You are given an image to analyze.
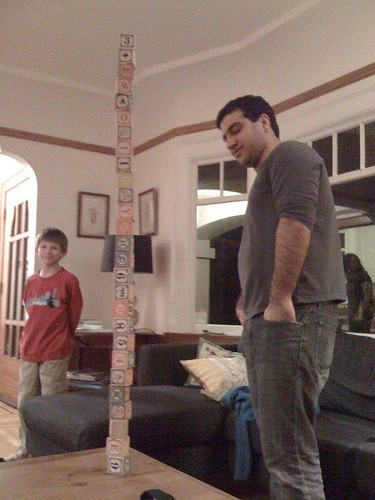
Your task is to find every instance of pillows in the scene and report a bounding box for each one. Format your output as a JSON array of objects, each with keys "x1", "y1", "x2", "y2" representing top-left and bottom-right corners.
[
  {"x1": 184, "y1": 336, "x2": 244, "y2": 387},
  {"x1": 179, "y1": 357, "x2": 249, "y2": 401}
]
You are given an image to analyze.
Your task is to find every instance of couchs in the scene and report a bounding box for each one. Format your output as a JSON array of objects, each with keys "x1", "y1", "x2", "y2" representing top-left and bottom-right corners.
[{"x1": 20, "y1": 330, "x2": 375, "y2": 500}]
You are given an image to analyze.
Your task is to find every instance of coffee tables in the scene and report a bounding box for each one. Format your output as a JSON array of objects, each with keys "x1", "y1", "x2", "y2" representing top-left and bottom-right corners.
[{"x1": 0, "y1": 446, "x2": 243, "y2": 500}]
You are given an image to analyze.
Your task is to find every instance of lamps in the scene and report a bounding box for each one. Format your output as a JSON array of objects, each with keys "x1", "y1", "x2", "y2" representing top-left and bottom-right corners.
[{"x1": 99, "y1": 236, "x2": 153, "y2": 325}]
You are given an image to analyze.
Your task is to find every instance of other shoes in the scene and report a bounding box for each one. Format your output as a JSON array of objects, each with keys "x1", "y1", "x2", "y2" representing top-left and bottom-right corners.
[{"x1": 0, "y1": 451, "x2": 28, "y2": 462}]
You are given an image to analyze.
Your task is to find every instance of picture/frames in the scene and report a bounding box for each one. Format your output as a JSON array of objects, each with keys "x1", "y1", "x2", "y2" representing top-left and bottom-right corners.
[
  {"x1": 138, "y1": 187, "x2": 159, "y2": 237},
  {"x1": 77, "y1": 191, "x2": 111, "y2": 239}
]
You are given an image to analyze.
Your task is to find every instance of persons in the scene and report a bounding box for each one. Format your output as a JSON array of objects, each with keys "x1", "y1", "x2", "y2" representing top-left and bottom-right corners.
[
  {"x1": 0, "y1": 227, "x2": 84, "y2": 462},
  {"x1": 216, "y1": 95, "x2": 375, "y2": 500}
]
